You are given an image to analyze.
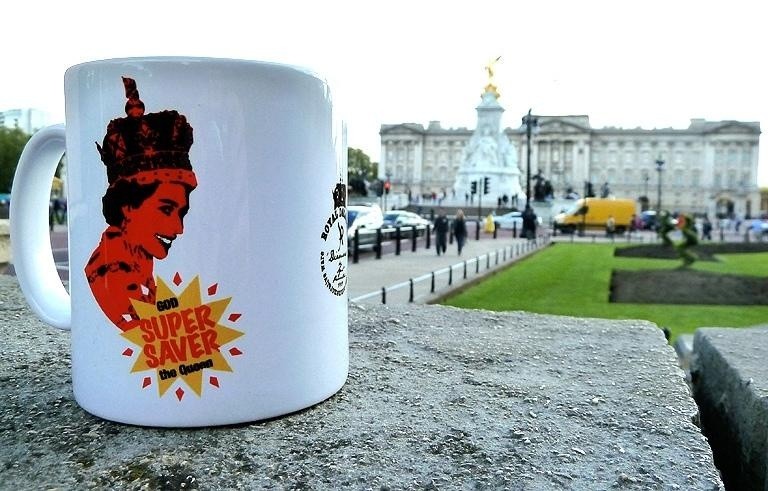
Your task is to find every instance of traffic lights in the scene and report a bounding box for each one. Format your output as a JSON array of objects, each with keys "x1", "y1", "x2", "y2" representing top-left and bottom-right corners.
[
  {"x1": 384, "y1": 180, "x2": 391, "y2": 194},
  {"x1": 471, "y1": 180, "x2": 477, "y2": 193},
  {"x1": 484, "y1": 177, "x2": 491, "y2": 194}
]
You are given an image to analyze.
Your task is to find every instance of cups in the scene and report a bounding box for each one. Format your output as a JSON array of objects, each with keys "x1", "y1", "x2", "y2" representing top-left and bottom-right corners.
[{"x1": 1, "y1": 50, "x2": 353, "y2": 432}]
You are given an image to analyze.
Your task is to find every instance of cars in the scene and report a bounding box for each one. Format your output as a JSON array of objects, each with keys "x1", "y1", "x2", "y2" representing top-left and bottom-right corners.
[
  {"x1": 640, "y1": 210, "x2": 674, "y2": 231},
  {"x1": 484, "y1": 210, "x2": 542, "y2": 230},
  {"x1": 384, "y1": 211, "x2": 431, "y2": 240}
]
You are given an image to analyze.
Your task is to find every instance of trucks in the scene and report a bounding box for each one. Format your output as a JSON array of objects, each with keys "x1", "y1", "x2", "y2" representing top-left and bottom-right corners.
[{"x1": 552, "y1": 197, "x2": 636, "y2": 234}]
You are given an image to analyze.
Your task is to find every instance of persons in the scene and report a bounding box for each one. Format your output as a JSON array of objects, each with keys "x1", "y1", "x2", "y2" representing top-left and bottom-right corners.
[
  {"x1": 452, "y1": 207, "x2": 468, "y2": 257},
  {"x1": 83, "y1": 77, "x2": 200, "y2": 335},
  {"x1": 430, "y1": 206, "x2": 449, "y2": 256},
  {"x1": 552, "y1": 208, "x2": 767, "y2": 244},
  {"x1": 518, "y1": 202, "x2": 536, "y2": 243},
  {"x1": 483, "y1": 208, "x2": 496, "y2": 237}
]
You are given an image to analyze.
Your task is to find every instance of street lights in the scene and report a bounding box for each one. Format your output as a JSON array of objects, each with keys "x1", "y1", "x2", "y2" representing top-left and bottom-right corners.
[
  {"x1": 519, "y1": 110, "x2": 541, "y2": 237},
  {"x1": 654, "y1": 154, "x2": 663, "y2": 210}
]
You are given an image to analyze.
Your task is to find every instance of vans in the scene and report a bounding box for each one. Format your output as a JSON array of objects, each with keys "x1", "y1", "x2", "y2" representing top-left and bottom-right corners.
[{"x1": 347, "y1": 203, "x2": 384, "y2": 255}]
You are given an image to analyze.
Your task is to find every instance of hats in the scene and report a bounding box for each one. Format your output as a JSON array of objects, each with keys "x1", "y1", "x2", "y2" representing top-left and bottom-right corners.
[{"x1": 103, "y1": 109, "x2": 199, "y2": 190}]
